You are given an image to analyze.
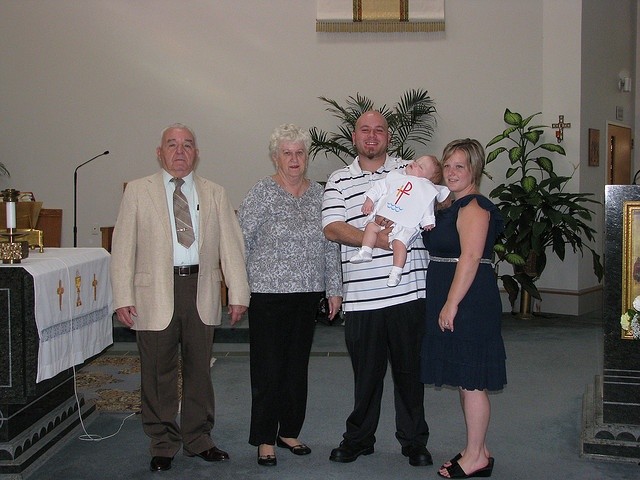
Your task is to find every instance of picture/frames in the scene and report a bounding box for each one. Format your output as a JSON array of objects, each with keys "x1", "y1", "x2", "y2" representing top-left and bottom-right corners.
[{"x1": 588, "y1": 127, "x2": 600, "y2": 167}]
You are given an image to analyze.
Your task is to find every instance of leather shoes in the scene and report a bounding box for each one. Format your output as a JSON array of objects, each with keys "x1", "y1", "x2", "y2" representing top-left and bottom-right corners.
[
  {"x1": 400, "y1": 443, "x2": 434, "y2": 468},
  {"x1": 329, "y1": 436, "x2": 377, "y2": 464},
  {"x1": 276, "y1": 434, "x2": 312, "y2": 456},
  {"x1": 149, "y1": 455, "x2": 174, "y2": 471},
  {"x1": 256, "y1": 443, "x2": 277, "y2": 467},
  {"x1": 181, "y1": 438, "x2": 229, "y2": 462}
]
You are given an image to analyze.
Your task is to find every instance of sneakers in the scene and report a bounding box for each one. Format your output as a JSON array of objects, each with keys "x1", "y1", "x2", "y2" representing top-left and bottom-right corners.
[
  {"x1": 350, "y1": 246, "x2": 374, "y2": 264},
  {"x1": 386, "y1": 266, "x2": 403, "y2": 287}
]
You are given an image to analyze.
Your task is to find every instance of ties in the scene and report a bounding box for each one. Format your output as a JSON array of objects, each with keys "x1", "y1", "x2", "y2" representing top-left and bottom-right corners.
[{"x1": 167, "y1": 177, "x2": 195, "y2": 248}]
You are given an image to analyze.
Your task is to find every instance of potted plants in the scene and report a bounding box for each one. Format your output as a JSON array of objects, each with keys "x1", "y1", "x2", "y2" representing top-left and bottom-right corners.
[{"x1": 486, "y1": 108, "x2": 605, "y2": 310}]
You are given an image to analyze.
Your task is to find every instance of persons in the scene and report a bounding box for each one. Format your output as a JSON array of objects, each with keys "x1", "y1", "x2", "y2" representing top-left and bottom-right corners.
[
  {"x1": 418, "y1": 136, "x2": 509, "y2": 480},
  {"x1": 108, "y1": 124, "x2": 252, "y2": 473},
  {"x1": 321, "y1": 109, "x2": 440, "y2": 467},
  {"x1": 348, "y1": 154, "x2": 442, "y2": 288},
  {"x1": 235, "y1": 121, "x2": 345, "y2": 467}
]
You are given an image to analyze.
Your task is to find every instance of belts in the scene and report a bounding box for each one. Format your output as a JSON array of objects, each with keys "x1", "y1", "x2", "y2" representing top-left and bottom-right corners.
[{"x1": 173, "y1": 263, "x2": 200, "y2": 278}]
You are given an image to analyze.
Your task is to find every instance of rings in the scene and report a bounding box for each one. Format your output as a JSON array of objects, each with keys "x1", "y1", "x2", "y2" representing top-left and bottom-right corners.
[{"x1": 337, "y1": 307, "x2": 341, "y2": 312}]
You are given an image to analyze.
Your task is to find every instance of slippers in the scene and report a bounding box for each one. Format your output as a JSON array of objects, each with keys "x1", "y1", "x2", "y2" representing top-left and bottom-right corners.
[
  {"x1": 441, "y1": 450, "x2": 494, "y2": 469},
  {"x1": 436, "y1": 459, "x2": 493, "y2": 477}
]
